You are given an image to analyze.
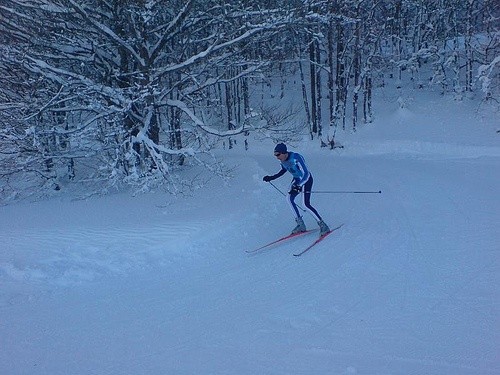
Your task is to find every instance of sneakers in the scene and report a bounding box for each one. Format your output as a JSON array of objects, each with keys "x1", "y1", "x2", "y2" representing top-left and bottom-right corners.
[
  {"x1": 318, "y1": 219, "x2": 331, "y2": 236},
  {"x1": 291, "y1": 217, "x2": 306, "y2": 234}
]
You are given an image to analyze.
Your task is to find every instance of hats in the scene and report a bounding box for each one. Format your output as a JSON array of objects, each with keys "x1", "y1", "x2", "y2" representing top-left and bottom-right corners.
[{"x1": 274, "y1": 142, "x2": 287, "y2": 153}]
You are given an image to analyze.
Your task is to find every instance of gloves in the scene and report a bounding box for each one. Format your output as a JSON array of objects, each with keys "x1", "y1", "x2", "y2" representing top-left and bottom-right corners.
[
  {"x1": 289, "y1": 187, "x2": 300, "y2": 196},
  {"x1": 263, "y1": 175, "x2": 271, "y2": 182}
]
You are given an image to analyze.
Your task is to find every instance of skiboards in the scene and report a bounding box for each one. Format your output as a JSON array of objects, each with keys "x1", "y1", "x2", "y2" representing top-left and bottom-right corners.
[{"x1": 244, "y1": 223, "x2": 345, "y2": 257}]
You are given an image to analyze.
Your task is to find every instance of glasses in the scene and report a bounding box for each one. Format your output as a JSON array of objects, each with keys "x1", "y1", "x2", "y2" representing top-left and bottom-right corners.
[{"x1": 275, "y1": 153, "x2": 282, "y2": 157}]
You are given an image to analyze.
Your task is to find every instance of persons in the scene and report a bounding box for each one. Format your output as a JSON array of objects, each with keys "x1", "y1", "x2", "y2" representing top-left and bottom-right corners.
[{"x1": 262, "y1": 142, "x2": 331, "y2": 239}]
